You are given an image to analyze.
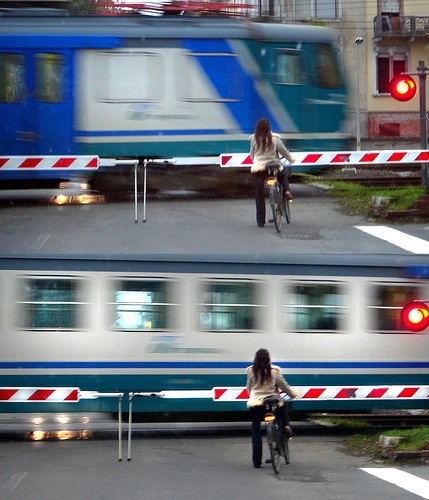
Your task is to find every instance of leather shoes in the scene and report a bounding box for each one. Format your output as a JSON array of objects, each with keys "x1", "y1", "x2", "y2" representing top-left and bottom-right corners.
[
  {"x1": 286, "y1": 194, "x2": 293, "y2": 199},
  {"x1": 285, "y1": 426, "x2": 293, "y2": 437},
  {"x1": 258, "y1": 222, "x2": 263, "y2": 227},
  {"x1": 254, "y1": 462, "x2": 261, "y2": 468}
]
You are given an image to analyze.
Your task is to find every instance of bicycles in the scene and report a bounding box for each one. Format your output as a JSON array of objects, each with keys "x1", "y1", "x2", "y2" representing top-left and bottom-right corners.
[
  {"x1": 264, "y1": 156, "x2": 291, "y2": 232},
  {"x1": 264, "y1": 388, "x2": 291, "y2": 474}
]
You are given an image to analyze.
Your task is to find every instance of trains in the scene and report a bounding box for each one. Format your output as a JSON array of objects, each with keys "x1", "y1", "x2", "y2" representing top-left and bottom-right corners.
[
  {"x1": 1, "y1": 1, "x2": 352, "y2": 197},
  {"x1": 0, "y1": 247, "x2": 429, "y2": 431}
]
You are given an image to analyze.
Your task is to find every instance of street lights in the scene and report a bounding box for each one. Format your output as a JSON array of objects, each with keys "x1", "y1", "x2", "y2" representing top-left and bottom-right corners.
[{"x1": 354, "y1": 37, "x2": 364, "y2": 152}]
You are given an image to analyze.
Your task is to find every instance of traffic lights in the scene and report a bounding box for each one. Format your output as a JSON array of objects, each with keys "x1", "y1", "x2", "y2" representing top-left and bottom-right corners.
[
  {"x1": 390, "y1": 73, "x2": 416, "y2": 102},
  {"x1": 402, "y1": 302, "x2": 428, "y2": 331}
]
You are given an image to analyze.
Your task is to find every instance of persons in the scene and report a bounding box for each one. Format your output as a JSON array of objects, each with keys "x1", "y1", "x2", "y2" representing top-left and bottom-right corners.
[
  {"x1": 246, "y1": 349, "x2": 297, "y2": 468},
  {"x1": 249, "y1": 118, "x2": 296, "y2": 227}
]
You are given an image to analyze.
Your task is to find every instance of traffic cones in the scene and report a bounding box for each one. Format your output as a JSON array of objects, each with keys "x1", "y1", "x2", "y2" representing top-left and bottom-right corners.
[
  {"x1": 0, "y1": 384, "x2": 429, "y2": 463},
  {"x1": 0, "y1": 150, "x2": 429, "y2": 222}
]
